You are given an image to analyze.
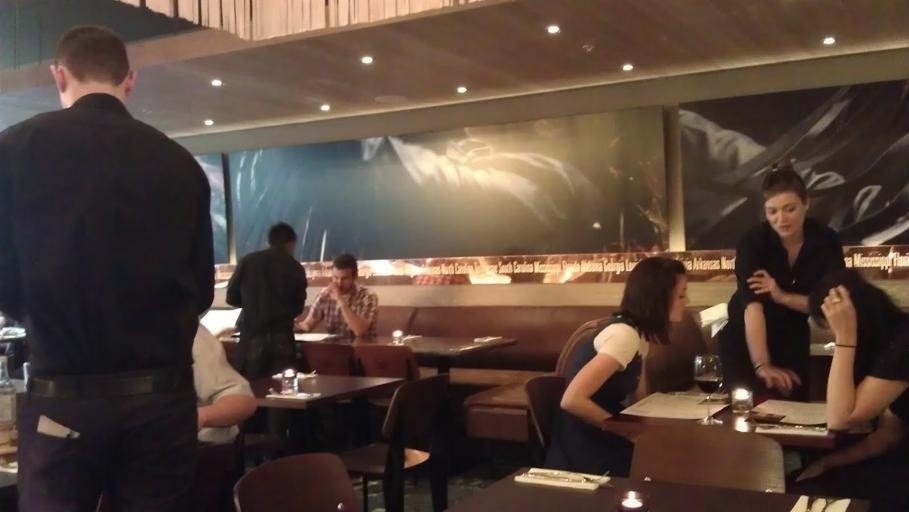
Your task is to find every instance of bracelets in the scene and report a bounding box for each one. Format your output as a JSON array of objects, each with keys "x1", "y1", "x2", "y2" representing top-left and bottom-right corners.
[
  {"x1": 752, "y1": 360, "x2": 770, "y2": 372},
  {"x1": 835, "y1": 342, "x2": 857, "y2": 349}
]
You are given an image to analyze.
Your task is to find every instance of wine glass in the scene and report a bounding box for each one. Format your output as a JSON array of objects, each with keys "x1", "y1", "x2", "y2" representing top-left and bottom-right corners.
[{"x1": 693, "y1": 353, "x2": 728, "y2": 429}]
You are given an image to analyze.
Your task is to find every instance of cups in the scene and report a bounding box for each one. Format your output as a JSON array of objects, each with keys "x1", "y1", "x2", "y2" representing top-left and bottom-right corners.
[
  {"x1": 613, "y1": 491, "x2": 648, "y2": 511},
  {"x1": 731, "y1": 390, "x2": 755, "y2": 416},
  {"x1": 280, "y1": 372, "x2": 299, "y2": 396}
]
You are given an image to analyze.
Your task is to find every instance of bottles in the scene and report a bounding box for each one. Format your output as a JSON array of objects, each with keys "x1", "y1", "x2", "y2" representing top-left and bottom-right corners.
[{"x1": 0, "y1": 356, "x2": 20, "y2": 469}]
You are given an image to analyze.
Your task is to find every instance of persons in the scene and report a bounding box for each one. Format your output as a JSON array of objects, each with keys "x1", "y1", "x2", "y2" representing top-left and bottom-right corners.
[
  {"x1": 226, "y1": 221, "x2": 308, "y2": 454},
  {"x1": 0, "y1": 24, "x2": 215, "y2": 511},
  {"x1": 193, "y1": 316, "x2": 256, "y2": 447},
  {"x1": 717, "y1": 162, "x2": 844, "y2": 393},
  {"x1": 785, "y1": 270, "x2": 905, "y2": 498},
  {"x1": 292, "y1": 254, "x2": 378, "y2": 337},
  {"x1": 557, "y1": 252, "x2": 688, "y2": 472}
]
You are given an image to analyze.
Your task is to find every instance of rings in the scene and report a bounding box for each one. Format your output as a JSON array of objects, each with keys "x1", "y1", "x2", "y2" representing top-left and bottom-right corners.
[{"x1": 831, "y1": 298, "x2": 840, "y2": 303}]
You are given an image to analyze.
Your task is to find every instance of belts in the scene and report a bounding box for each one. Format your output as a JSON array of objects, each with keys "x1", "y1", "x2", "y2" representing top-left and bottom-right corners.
[{"x1": 21, "y1": 371, "x2": 197, "y2": 401}]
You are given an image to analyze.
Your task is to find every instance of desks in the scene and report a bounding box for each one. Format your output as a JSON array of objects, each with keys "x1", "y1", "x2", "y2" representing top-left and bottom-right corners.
[{"x1": 0, "y1": 301, "x2": 909, "y2": 512}]
